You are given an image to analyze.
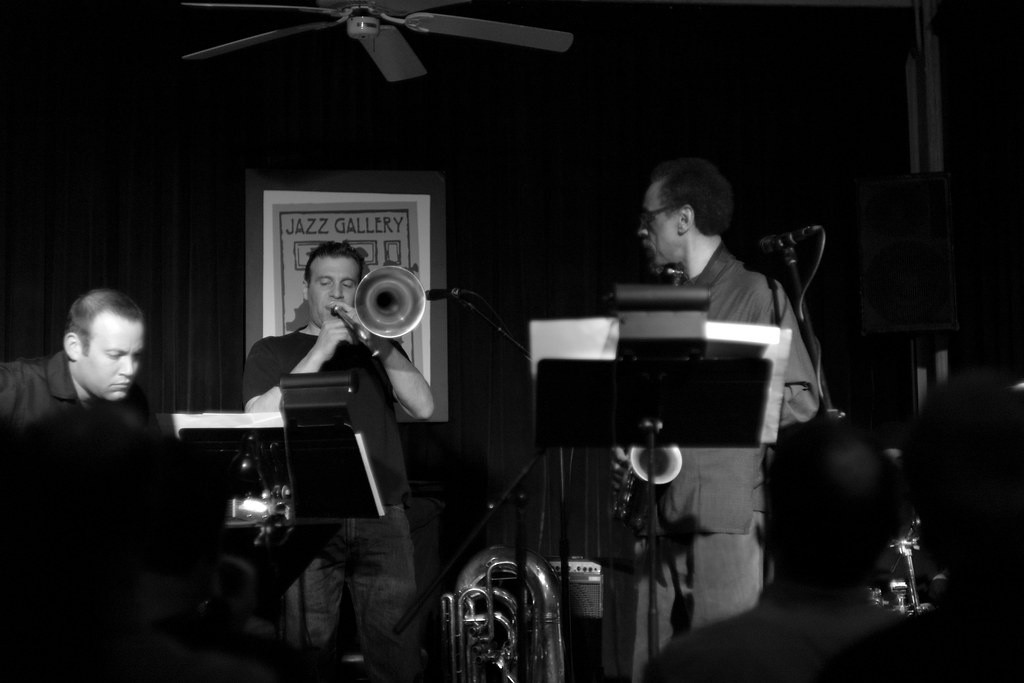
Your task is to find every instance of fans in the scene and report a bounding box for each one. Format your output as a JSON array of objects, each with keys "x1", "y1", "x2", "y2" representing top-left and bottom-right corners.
[{"x1": 178, "y1": 0, "x2": 575, "y2": 83}]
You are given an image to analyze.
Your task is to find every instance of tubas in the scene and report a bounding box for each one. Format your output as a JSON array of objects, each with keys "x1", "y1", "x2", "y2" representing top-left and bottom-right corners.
[{"x1": 439, "y1": 543, "x2": 568, "y2": 683}]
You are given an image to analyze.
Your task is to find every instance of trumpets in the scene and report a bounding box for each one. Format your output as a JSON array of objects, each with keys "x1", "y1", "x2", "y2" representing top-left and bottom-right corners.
[{"x1": 325, "y1": 263, "x2": 427, "y2": 358}]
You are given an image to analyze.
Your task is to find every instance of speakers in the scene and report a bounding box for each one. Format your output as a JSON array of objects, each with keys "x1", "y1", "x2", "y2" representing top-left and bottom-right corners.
[{"x1": 537, "y1": 556, "x2": 605, "y2": 683}]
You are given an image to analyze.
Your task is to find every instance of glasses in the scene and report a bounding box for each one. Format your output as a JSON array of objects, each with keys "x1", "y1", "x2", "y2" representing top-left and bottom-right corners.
[{"x1": 635, "y1": 203, "x2": 678, "y2": 231}]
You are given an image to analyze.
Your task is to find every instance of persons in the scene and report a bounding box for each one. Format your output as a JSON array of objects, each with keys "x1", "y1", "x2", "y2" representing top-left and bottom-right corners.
[
  {"x1": 242, "y1": 243, "x2": 435, "y2": 683},
  {"x1": 0, "y1": 288, "x2": 307, "y2": 683},
  {"x1": 813, "y1": 368, "x2": 1024, "y2": 683},
  {"x1": 639, "y1": 419, "x2": 908, "y2": 683},
  {"x1": 602, "y1": 161, "x2": 820, "y2": 683}
]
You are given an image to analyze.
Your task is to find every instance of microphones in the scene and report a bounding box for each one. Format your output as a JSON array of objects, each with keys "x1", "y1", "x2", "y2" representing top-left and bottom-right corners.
[
  {"x1": 424, "y1": 289, "x2": 463, "y2": 301},
  {"x1": 759, "y1": 225, "x2": 821, "y2": 253}
]
